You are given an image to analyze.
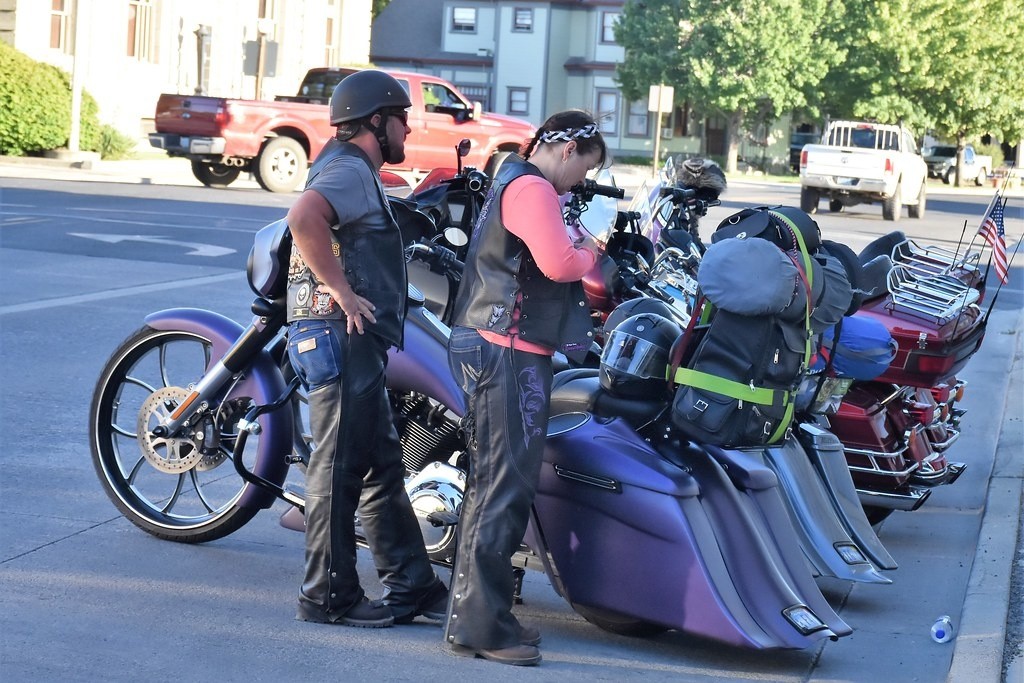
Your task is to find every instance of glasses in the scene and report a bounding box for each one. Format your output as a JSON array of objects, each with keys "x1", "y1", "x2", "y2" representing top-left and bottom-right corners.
[{"x1": 377, "y1": 107, "x2": 408, "y2": 122}]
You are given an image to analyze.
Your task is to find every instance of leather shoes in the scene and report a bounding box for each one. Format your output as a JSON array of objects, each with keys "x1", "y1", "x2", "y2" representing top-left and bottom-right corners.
[
  {"x1": 294, "y1": 595, "x2": 394, "y2": 627},
  {"x1": 513, "y1": 622, "x2": 543, "y2": 645},
  {"x1": 394, "y1": 589, "x2": 452, "y2": 623},
  {"x1": 450, "y1": 641, "x2": 543, "y2": 666}
]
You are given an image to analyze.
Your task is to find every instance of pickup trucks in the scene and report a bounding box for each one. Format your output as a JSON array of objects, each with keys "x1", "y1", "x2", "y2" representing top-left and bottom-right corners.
[
  {"x1": 798, "y1": 121, "x2": 928, "y2": 221},
  {"x1": 923, "y1": 143, "x2": 993, "y2": 185},
  {"x1": 149, "y1": 66, "x2": 539, "y2": 194}
]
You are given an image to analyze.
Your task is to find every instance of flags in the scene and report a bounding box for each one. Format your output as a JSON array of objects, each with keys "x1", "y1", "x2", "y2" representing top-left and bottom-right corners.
[{"x1": 979, "y1": 197, "x2": 1009, "y2": 287}]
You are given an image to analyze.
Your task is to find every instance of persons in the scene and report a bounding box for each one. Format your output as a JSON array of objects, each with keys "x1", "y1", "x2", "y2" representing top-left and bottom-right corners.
[
  {"x1": 444, "y1": 110, "x2": 608, "y2": 666},
  {"x1": 285, "y1": 68, "x2": 450, "y2": 628}
]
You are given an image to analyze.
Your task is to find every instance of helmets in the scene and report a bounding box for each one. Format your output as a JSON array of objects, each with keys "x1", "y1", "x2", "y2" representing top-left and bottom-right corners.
[
  {"x1": 598, "y1": 297, "x2": 684, "y2": 402},
  {"x1": 328, "y1": 68, "x2": 413, "y2": 127}
]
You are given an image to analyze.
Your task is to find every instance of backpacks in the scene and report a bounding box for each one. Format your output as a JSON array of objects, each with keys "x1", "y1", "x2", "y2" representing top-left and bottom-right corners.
[{"x1": 667, "y1": 204, "x2": 901, "y2": 452}]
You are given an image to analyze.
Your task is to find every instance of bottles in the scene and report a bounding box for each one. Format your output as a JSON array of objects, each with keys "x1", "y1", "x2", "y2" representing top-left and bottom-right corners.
[{"x1": 930, "y1": 616, "x2": 954, "y2": 643}]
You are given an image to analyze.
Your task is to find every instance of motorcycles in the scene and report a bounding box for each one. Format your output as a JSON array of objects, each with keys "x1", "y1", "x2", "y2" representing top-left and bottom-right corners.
[{"x1": 89, "y1": 139, "x2": 984, "y2": 653}]
